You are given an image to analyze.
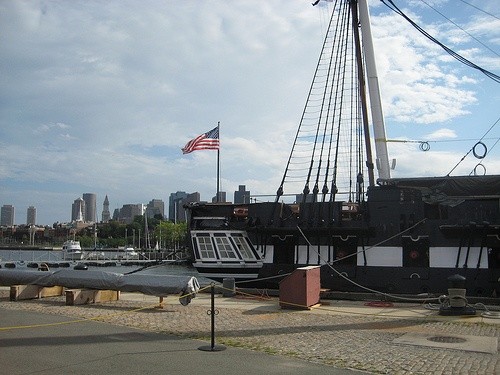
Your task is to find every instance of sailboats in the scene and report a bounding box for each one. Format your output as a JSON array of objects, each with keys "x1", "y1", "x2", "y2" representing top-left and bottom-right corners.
[
  {"x1": 62, "y1": 234, "x2": 84, "y2": 260},
  {"x1": 183, "y1": 0, "x2": 500, "y2": 302}
]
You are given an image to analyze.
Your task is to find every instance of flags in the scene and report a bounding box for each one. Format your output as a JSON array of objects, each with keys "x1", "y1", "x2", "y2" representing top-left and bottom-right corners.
[{"x1": 181, "y1": 127, "x2": 220, "y2": 155}]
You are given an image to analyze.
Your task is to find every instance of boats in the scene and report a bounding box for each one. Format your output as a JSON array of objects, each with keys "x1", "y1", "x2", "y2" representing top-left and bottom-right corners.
[{"x1": 117, "y1": 247, "x2": 138, "y2": 257}]
